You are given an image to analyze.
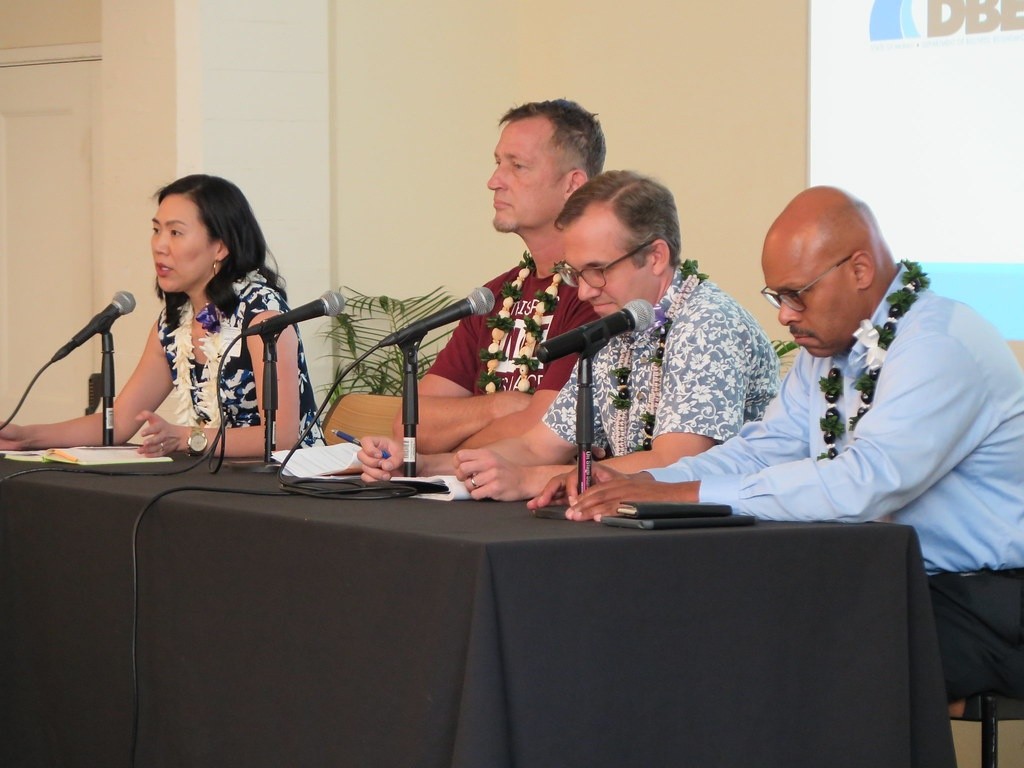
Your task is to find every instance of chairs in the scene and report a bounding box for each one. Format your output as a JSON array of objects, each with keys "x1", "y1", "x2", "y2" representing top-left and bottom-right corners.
[{"x1": 320, "y1": 395, "x2": 403, "y2": 447}]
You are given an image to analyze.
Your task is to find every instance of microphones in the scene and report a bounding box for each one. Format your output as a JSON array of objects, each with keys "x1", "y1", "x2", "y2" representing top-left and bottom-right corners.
[
  {"x1": 537, "y1": 299, "x2": 656, "y2": 364},
  {"x1": 378, "y1": 287, "x2": 495, "y2": 348},
  {"x1": 242, "y1": 290, "x2": 344, "y2": 337},
  {"x1": 51, "y1": 291, "x2": 136, "y2": 362}
]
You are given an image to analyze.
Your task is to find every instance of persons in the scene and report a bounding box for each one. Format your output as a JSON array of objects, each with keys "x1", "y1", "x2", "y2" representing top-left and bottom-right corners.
[
  {"x1": 0, "y1": 173, "x2": 325, "y2": 458},
  {"x1": 525, "y1": 185, "x2": 1024, "y2": 707},
  {"x1": 394, "y1": 99, "x2": 606, "y2": 455},
  {"x1": 356, "y1": 170, "x2": 782, "y2": 502}
]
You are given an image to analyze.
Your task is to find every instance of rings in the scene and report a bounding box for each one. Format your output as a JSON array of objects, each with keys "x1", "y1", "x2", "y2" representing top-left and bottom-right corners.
[
  {"x1": 161, "y1": 442, "x2": 164, "y2": 451},
  {"x1": 471, "y1": 474, "x2": 481, "y2": 488}
]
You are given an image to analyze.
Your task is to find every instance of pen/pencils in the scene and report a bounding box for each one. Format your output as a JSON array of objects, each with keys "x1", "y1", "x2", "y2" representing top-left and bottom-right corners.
[{"x1": 329, "y1": 429, "x2": 390, "y2": 459}]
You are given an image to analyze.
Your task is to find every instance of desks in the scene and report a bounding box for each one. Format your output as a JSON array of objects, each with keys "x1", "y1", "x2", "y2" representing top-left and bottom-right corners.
[{"x1": 0, "y1": 443, "x2": 958, "y2": 768}]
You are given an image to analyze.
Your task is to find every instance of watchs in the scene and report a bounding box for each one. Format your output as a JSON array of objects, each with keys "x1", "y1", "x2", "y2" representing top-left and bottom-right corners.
[{"x1": 185, "y1": 425, "x2": 208, "y2": 456}]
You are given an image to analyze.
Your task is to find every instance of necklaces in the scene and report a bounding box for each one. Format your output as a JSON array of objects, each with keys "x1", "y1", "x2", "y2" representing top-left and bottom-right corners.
[
  {"x1": 476, "y1": 250, "x2": 565, "y2": 394},
  {"x1": 817, "y1": 260, "x2": 930, "y2": 463},
  {"x1": 611, "y1": 257, "x2": 710, "y2": 457},
  {"x1": 174, "y1": 268, "x2": 267, "y2": 429}
]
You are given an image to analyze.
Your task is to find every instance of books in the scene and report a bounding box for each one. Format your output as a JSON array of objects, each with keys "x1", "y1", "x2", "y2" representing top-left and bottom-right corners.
[
  {"x1": 616, "y1": 502, "x2": 733, "y2": 519},
  {"x1": 3, "y1": 444, "x2": 175, "y2": 467}
]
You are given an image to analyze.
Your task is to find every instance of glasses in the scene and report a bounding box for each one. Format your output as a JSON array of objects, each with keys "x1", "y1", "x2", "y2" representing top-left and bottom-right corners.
[
  {"x1": 761, "y1": 254, "x2": 852, "y2": 313},
  {"x1": 668, "y1": 310, "x2": 697, "y2": 328},
  {"x1": 556, "y1": 237, "x2": 659, "y2": 288}
]
